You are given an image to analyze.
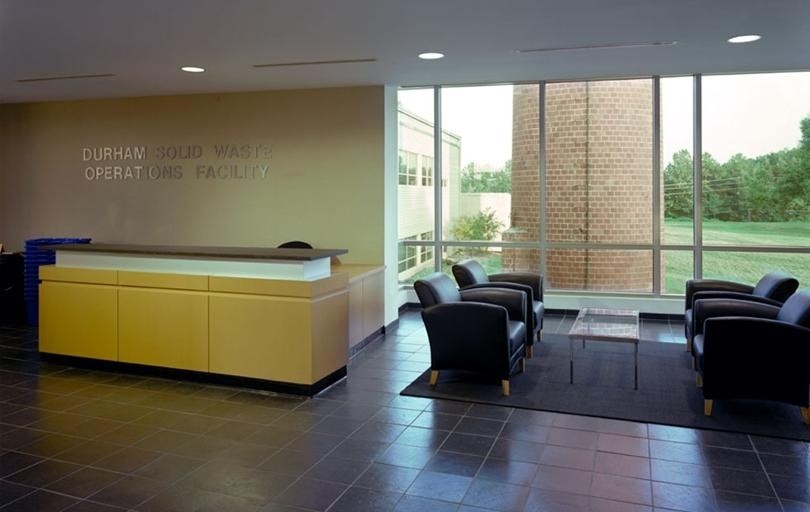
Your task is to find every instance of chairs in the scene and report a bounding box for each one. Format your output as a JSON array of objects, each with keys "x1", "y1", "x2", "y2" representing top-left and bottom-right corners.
[
  {"x1": 414, "y1": 273, "x2": 527, "y2": 396},
  {"x1": 685, "y1": 271, "x2": 799, "y2": 368},
  {"x1": 691, "y1": 291, "x2": 810, "y2": 424},
  {"x1": 451, "y1": 259, "x2": 545, "y2": 358}
]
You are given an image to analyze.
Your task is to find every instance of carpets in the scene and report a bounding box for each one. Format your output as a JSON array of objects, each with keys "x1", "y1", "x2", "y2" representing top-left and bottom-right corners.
[{"x1": 400, "y1": 332, "x2": 810, "y2": 444}]
